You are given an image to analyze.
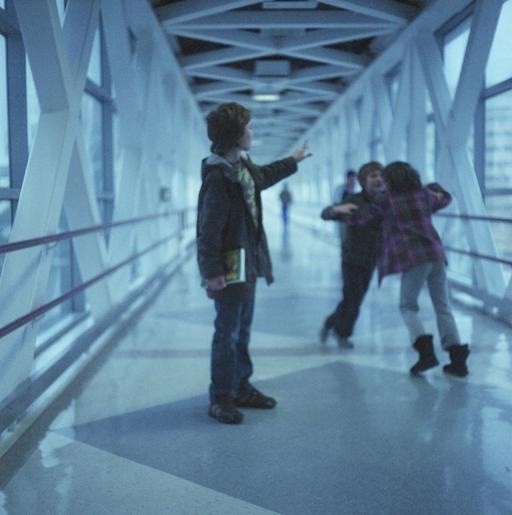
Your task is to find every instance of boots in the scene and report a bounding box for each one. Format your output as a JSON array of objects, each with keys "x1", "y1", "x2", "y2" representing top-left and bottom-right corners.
[
  {"x1": 410, "y1": 335, "x2": 439, "y2": 374},
  {"x1": 443, "y1": 344, "x2": 470, "y2": 377}
]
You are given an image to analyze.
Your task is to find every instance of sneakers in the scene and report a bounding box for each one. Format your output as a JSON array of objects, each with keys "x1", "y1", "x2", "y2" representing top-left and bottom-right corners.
[
  {"x1": 209, "y1": 403, "x2": 243, "y2": 423},
  {"x1": 337, "y1": 335, "x2": 354, "y2": 348},
  {"x1": 234, "y1": 382, "x2": 277, "y2": 409},
  {"x1": 321, "y1": 318, "x2": 332, "y2": 342}
]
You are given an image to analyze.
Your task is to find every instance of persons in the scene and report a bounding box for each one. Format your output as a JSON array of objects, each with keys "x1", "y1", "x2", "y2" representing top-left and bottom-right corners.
[
  {"x1": 338, "y1": 162, "x2": 471, "y2": 376},
  {"x1": 317, "y1": 161, "x2": 445, "y2": 349},
  {"x1": 195, "y1": 101, "x2": 314, "y2": 423},
  {"x1": 280, "y1": 183, "x2": 293, "y2": 220},
  {"x1": 332, "y1": 169, "x2": 356, "y2": 200}
]
